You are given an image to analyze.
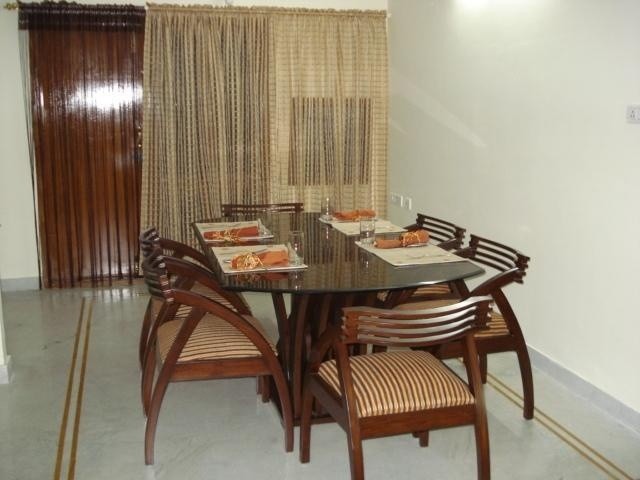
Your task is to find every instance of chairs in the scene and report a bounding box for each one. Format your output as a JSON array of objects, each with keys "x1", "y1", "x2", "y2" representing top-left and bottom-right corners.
[
  {"x1": 397, "y1": 233, "x2": 534, "y2": 440},
  {"x1": 139, "y1": 248, "x2": 296, "y2": 467},
  {"x1": 358, "y1": 213, "x2": 469, "y2": 310},
  {"x1": 300, "y1": 295, "x2": 503, "y2": 480},
  {"x1": 141, "y1": 226, "x2": 255, "y2": 375},
  {"x1": 222, "y1": 202, "x2": 305, "y2": 218}
]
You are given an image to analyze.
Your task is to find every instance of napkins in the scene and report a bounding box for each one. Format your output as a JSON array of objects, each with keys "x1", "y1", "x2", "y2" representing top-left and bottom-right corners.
[
  {"x1": 204, "y1": 223, "x2": 261, "y2": 242},
  {"x1": 371, "y1": 226, "x2": 434, "y2": 254},
  {"x1": 232, "y1": 245, "x2": 293, "y2": 272},
  {"x1": 331, "y1": 209, "x2": 379, "y2": 225}
]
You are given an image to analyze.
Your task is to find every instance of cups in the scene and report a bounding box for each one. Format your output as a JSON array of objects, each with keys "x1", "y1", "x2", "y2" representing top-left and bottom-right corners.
[
  {"x1": 358, "y1": 214, "x2": 377, "y2": 245},
  {"x1": 256, "y1": 208, "x2": 273, "y2": 237},
  {"x1": 319, "y1": 191, "x2": 334, "y2": 220},
  {"x1": 288, "y1": 230, "x2": 304, "y2": 266}
]
